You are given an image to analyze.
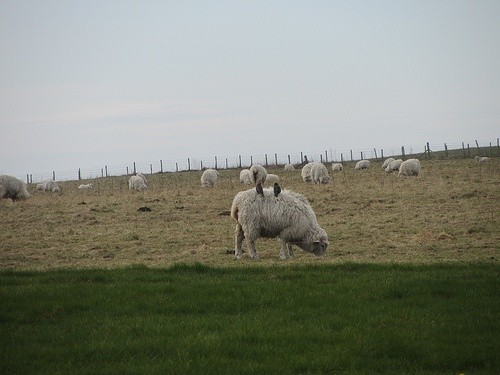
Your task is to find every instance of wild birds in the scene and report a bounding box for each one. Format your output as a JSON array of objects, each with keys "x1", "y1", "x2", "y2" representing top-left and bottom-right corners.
[
  {"x1": 256, "y1": 183, "x2": 264, "y2": 197},
  {"x1": 274, "y1": 183, "x2": 281, "y2": 197}
]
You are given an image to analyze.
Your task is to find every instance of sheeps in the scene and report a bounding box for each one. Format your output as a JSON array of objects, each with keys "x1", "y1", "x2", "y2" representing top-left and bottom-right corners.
[
  {"x1": 78, "y1": 183, "x2": 93, "y2": 189},
  {"x1": 301, "y1": 162, "x2": 331, "y2": 184},
  {"x1": 0, "y1": 174, "x2": 31, "y2": 203},
  {"x1": 240, "y1": 165, "x2": 280, "y2": 185},
  {"x1": 399, "y1": 159, "x2": 421, "y2": 176},
  {"x1": 382, "y1": 158, "x2": 403, "y2": 173},
  {"x1": 474, "y1": 156, "x2": 491, "y2": 163},
  {"x1": 201, "y1": 169, "x2": 222, "y2": 189},
  {"x1": 129, "y1": 176, "x2": 149, "y2": 191},
  {"x1": 332, "y1": 162, "x2": 343, "y2": 172},
  {"x1": 354, "y1": 160, "x2": 370, "y2": 170},
  {"x1": 230, "y1": 187, "x2": 330, "y2": 260},
  {"x1": 284, "y1": 164, "x2": 295, "y2": 170},
  {"x1": 36, "y1": 178, "x2": 62, "y2": 192}
]
